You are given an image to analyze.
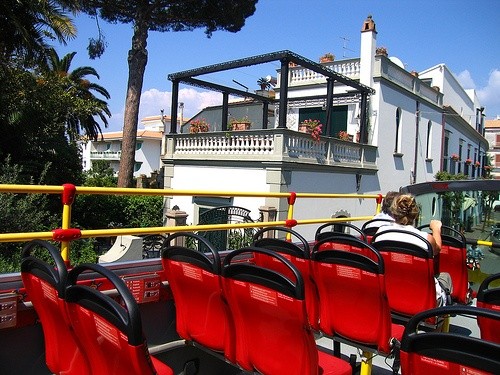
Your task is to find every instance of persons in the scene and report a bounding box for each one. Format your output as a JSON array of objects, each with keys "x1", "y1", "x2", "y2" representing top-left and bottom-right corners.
[
  {"x1": 375, "y1": 194, "x2": 453, "y2": 308},
  {"x1": 468, "y1": 244, "x2": 484, "y2": 258},
  {"x1": 369, "y1": 192, "x2": 399, "y2": 228}
]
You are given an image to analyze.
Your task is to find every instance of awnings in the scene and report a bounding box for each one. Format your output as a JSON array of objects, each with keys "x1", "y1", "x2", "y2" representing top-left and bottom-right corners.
[{"x1": 463, "y1": 197, "x2": 475, "y2": 211}]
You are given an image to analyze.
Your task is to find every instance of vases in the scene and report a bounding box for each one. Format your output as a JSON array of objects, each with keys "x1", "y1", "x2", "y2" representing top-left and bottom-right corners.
[
  {"x1": 377, "y1": 53, "x2": 388, "y2": 57},
  {"x1": 321, "y1": 58, "x2": 331, "y2": 63},
  {"x1": 232, "y1": 123, "x2": 250, "y2": 130},
  {"x1": 411, "y1": 72, "x2": 418, "y2": 77},
  {"x1": 433, "y1": 87, "x2": 439, "y2": 92}
]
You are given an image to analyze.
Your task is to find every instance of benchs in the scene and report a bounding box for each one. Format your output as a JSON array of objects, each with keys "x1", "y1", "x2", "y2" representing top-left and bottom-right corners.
[
  {"x1": 399, "y1": 307, "x2": 500, "y2": 375},
  {"x1": 314, "y1": 224, "x2": 457, "y2": 332},
  {"x1": 361, "y1": 219, "x2": 476, "y2": 305},
  {"x1": 162, "y1": 230, "x2": 356, "y2": 375},
  {"x1": 17, "y1": 239, "x2": 174, "y2": 375},
  {"x1": 250, "y1": 227, "x2": 407, "y2": 375},
  {"x1": 475, "y1": 273, "x2": 500, "y2": 346}
]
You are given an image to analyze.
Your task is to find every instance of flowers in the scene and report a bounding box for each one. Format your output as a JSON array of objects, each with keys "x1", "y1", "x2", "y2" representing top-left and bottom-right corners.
[
  {"x1": 450, "y1": 153, "x2": 494, "y2": 170},
  {"x1": 226, "y1": 116, "x2": 252, "y2": 138},
  {"x1": 319, "y1": 52, "x2": 335, "y2": 60},
  {"x1": 189, "y1": 118, "x2": 211, "y2": 134},
  {"x1": 376, "y1": 46, "x2": 387, "y2": 53},
  {"x1": 299, "y1": 119, "x2": 324, "y2": 141},
  {"x1": 338, "y1": 131, "x2": 349, "y2": 141}
]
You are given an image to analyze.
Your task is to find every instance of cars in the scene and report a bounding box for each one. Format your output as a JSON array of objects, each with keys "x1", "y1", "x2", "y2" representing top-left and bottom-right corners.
[{"x1": 489, "y1": 225, "x2": 500, "y2": 253}]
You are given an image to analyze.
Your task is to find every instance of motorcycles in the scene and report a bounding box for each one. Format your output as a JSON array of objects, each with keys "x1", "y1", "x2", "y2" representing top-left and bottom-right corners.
[{"x1": 465, "y1": 251, "x2": 484, "y2": 272}]
